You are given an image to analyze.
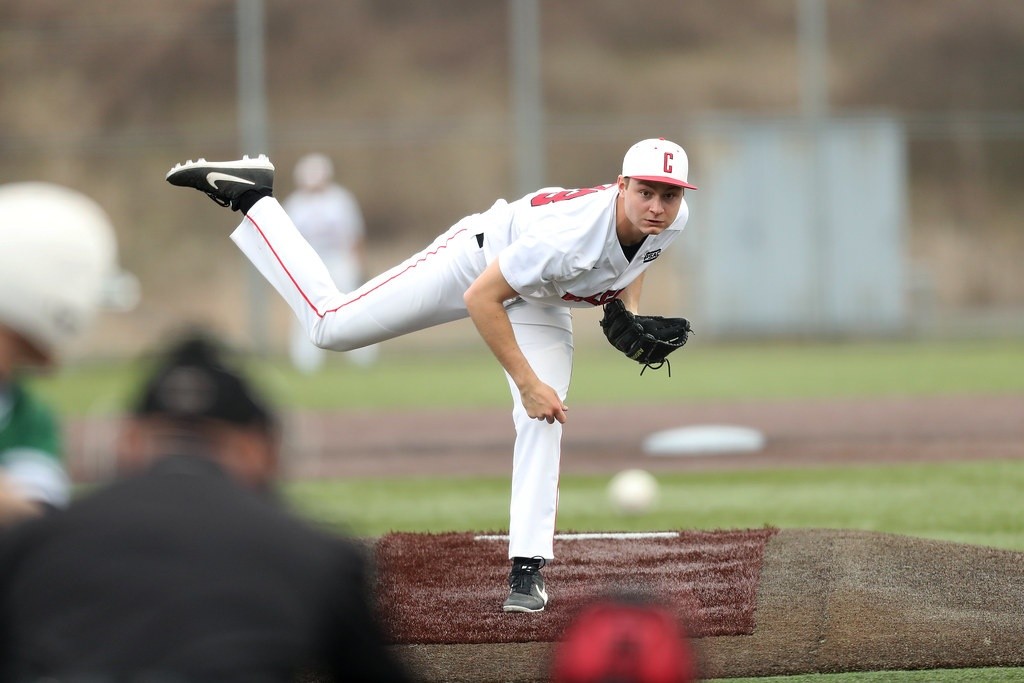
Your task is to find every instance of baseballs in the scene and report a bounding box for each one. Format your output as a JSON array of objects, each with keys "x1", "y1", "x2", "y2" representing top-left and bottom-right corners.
[{"x1": 606, "y1": 468, "x2": 664, "y2": 520}]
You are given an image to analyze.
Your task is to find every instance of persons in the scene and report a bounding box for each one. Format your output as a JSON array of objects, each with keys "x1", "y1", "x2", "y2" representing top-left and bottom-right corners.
[
  {"x1": 0, "y1": 182, "x2": 420, "y2": 683},
  {"x1": 545, "y1": 590, "x2": 701, "y2": 683},
  {"x1": 165, "y1": 137, "x2": 698, "y2": 612}
]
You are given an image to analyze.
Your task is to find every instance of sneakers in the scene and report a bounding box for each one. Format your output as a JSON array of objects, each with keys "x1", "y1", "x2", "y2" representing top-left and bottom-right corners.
[
  {"x1": 502, "y1": 555, "x2": 549, "y2": 613},
  {"x1": 164, "y1": 153, "x2": 275, "y2": 212}
]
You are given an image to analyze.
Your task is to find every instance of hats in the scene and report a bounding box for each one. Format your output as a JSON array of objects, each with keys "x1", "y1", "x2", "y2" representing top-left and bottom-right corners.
[
  {"x1": 0, "y1": 182, "x2": 119, "y2": 360},
  {"x1": 124, "y1": 323, "x2": 274, "y2": 426},
  {"x1": 622, "y1": 137, "x2": 698, "y2": 190}
]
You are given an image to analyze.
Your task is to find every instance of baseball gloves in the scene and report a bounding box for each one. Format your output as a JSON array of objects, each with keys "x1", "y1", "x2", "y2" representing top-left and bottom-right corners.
[{"x1": 599, "y1": 295, "x2": 696, "y2": 380}]
